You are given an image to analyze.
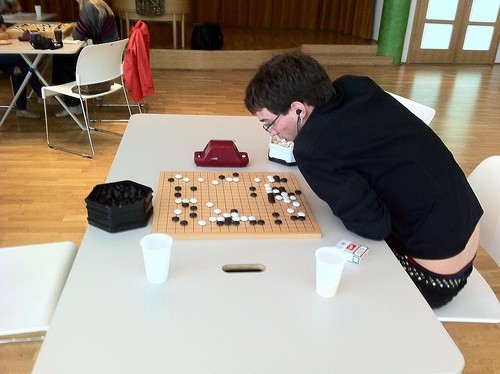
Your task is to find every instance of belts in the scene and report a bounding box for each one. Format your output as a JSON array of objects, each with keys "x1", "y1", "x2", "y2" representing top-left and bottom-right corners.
[{"x1": 394, "y1": 252, "x2": 473, "y2": 288}]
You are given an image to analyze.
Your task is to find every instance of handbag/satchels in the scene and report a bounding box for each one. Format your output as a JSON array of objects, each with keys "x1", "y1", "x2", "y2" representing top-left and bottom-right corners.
[{"x1": 71, "y1": 80, "x2": 114, "y2": 95}]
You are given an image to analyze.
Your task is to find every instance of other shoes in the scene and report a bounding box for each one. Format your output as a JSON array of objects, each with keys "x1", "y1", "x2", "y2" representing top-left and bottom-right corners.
[
  {"x1": 16, "y1": 110, "x2": 43, "y2": 119},
  {"x1": 55, "y1": 109, "x2": 82, "y2": 117},
  {"x1": 38, "y1": 97, "x2": 60, "y2": 106}
]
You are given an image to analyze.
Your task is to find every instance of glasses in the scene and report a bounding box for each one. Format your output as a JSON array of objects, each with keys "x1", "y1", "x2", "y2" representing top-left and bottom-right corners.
[{"x1": 262, "y1": 115, "x2": 279, "y2": 133}]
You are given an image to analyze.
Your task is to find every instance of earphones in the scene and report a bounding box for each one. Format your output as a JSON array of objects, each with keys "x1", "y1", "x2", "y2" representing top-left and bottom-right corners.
[{"x1": 296, "y1": 109, "x2": 302, "y2": 115}]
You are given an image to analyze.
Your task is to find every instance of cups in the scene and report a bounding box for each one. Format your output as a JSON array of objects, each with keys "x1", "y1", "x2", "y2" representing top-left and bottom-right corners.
[
  {"x1": 35, "y1": 5, "x2": 41, "y2": 17},
  {"x1": 315, "y1": 246, "x2": 347, "y2": 298},
  {"x1": 139, "y1": 233, "x2": 173, "y2": 284}
]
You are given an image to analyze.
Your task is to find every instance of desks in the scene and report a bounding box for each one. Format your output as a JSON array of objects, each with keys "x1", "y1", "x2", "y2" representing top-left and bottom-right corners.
[
  {"x1": 32, "y1": 113, "x2": 468, "y2": 374},
  {"x1": 0, "y1": 23, "x2": 88, "y2": 126},
  {"x1": 2, "y1": 13, "x2": 58, "y2": 24}
]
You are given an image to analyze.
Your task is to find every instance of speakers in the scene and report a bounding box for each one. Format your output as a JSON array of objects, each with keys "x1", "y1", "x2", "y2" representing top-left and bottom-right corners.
[{"x1": 191, "y1": 22, "x2": 224, "y2": 51}]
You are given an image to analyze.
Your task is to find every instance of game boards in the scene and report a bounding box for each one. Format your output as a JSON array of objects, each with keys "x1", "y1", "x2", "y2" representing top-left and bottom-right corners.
[
  {"x1": 150, "y1": 170, "x2": 323, "y2": 240},
  {"x1": 5, "y1": 23, "x2": 74, "y2": 40}
]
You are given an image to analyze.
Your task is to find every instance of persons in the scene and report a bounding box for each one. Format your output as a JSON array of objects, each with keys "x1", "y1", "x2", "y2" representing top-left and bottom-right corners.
[
  {"x1": 52, "y1": 0, "x2": 120, "y2": 117},
  {"x1": 0, "y1": 16, "x2": 65, "y2": 119},
  {"x1": 244, "y1": 50, "x2": 484, "y2": 309},
  {"x1": 0, "y1": 0, "x2": 21, "y2": 15}
]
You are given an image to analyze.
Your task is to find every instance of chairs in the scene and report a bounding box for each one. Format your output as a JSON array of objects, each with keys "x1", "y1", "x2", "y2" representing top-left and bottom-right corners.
[
  {"x1": 41, "y1": 38, "x2": 132, "y2": 159},
  {"x1": 85, "y1": 21, "x2": 154, "y2": 122},
  {"x1": 0, "y1": 240, "x2": 79, "y2": 347},
  {"x1": 434, "y1": 155, "x2": 500, "y2": 327}
]
